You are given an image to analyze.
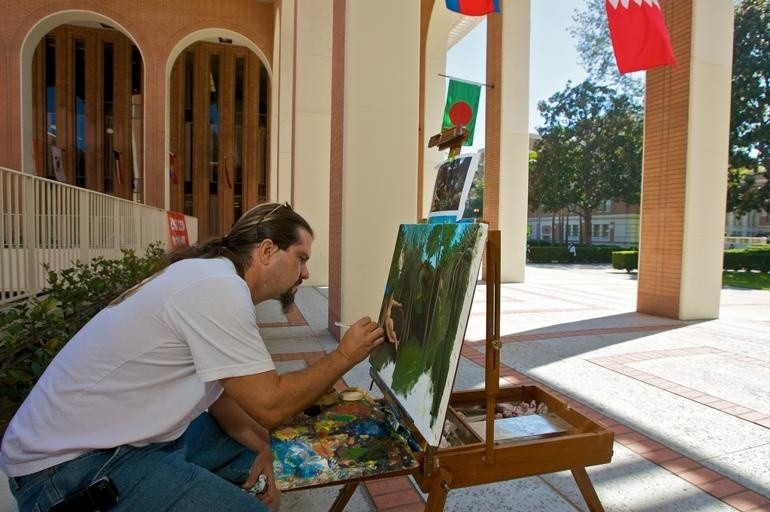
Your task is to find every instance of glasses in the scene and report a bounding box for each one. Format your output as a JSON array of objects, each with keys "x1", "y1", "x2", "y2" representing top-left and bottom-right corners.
[{"x1": 256, "y1": 201, "x2": 291, "y2": 246}]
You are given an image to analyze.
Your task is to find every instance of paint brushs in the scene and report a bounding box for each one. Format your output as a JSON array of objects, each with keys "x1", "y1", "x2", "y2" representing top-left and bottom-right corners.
[{"x1": 334, "y1": 320, "x2": 354, "y2": 330}]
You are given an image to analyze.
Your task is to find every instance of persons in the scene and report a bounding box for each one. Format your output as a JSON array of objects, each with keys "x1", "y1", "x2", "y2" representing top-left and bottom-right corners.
[{"x1": 2, "y1": 202, "x2": 385, "y2": 510}]
